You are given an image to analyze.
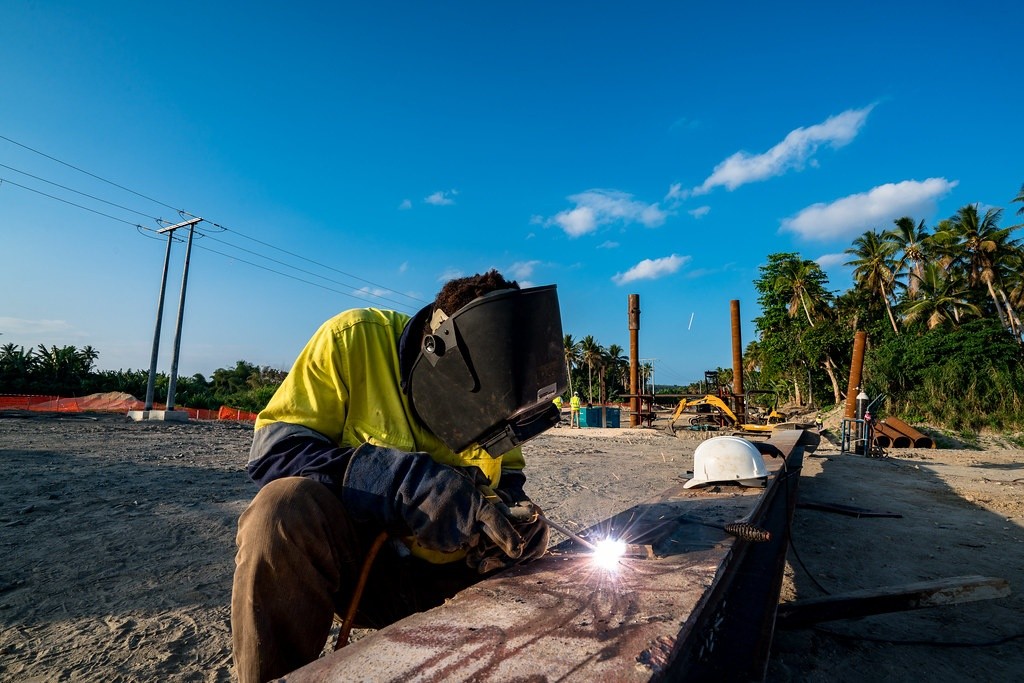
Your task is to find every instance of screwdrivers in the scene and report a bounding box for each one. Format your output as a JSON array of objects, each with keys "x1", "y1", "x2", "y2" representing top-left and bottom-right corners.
[{"x1": 675, "y1": 517, "x2": 770, "y2": 544}]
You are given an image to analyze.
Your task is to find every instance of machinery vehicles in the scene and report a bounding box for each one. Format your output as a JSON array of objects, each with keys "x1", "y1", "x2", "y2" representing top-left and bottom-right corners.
[{"x1": 663, "y1": 390, "x2": 788, "y2": 441}]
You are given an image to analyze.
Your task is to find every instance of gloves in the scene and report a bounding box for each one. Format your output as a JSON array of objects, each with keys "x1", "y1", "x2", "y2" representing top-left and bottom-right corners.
[{"x1": 342, "y1": 441, "x2": 549, "y2": 575}]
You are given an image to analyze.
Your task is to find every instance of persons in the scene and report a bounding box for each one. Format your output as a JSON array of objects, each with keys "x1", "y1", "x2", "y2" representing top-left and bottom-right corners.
[
  {"x1": 570, "y1": 391, "x2": 581, "y2": 429},
  {"x1": 552, "y1": 396, "x2": 564, "y2": 428},
  {"x1": 230, "y1": 268, "x2": 562, "y2": 683}
]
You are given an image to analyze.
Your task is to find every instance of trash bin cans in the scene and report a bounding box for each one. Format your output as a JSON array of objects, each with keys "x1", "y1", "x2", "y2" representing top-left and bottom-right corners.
[{"x1": 577, "y1": 405, "x2": 621, "y2": 429}]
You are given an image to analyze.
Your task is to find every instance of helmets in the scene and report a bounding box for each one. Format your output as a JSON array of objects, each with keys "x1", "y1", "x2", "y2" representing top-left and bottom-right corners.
[{"x1": 683, "y1": 436, "x2": 772, "y2": 488}]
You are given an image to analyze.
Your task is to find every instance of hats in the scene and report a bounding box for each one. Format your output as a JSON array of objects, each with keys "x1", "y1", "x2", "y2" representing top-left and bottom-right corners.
[
  {"x1": 574, "y1": 392, "x2": 578, "y2": 394},
  {"x1": 818, "y1": 415, "x2": 820, "y2": 416}
]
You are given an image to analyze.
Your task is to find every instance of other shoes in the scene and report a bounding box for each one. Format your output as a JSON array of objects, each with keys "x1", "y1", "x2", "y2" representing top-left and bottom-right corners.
[
  {"x1": 557, "y1": 426, "x2": 561, "y2": 428},
  {"x1": 554, "y1": 427, "x2": 556, "y2": 428}
]
